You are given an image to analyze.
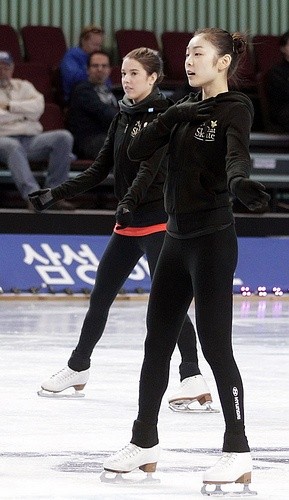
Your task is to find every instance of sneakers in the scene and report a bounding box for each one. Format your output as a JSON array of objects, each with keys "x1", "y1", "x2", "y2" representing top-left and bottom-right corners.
[
  {"x1": 100, "y1": 442, "x2": 160, "y2": 484},
  {"x1": 49, "y1": 199, "x2": 75, "y2": 211},
  {"x1": 201, "y1": 452, "x2": 258, "y2": 497},
  {"x1": 168, "y1": 375, "x2": 220, "y2": 413},
  {"x1": 37, "y1": 365, "x2": 89, "y2": 399}
]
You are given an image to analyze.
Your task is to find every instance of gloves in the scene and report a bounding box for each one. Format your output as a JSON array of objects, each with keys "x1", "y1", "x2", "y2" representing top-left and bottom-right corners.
[
  {"x1": 28, "y1": 187, "x2": 56, "y2": 212},
  {"x1": 115, "y1": 204, "x2": 131, "y2": 230},
  {"x1": 230, "y1": 177, "x2": 271, "y2": 212},
  {"x1": 177, "y1": 96, "x2": 215, "y2": 119}
]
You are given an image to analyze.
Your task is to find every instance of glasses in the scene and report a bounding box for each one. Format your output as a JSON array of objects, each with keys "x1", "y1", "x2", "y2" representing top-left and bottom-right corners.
[{"x1": 83, "y1": 28, "x2": 106, "y2": 35}]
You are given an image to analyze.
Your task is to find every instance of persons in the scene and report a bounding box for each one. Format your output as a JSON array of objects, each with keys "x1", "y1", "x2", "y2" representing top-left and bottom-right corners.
[
  {"x1": 265, "y1": 28, "x2": 289, "y2": 132},
  {"x1": 66, "y1": 52, "x2": 119, "y2": 159},
  {"x1": 0, "y1": 51, "x2": 79, "y2": 211},
  {"x1": 99, "y1": 28, "x2": 271, "y2": 496},
  {"x1": 27, "y1": 47, "x2": 218, "y2": 413},
  {"x1": 59, "y1": 26, "x2": 125, "y2": 112}
]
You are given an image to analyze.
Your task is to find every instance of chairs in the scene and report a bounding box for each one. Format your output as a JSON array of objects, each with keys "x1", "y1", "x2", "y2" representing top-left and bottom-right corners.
[{"x1": 0, "y1": 25, "x2": 289, "y2": 169}]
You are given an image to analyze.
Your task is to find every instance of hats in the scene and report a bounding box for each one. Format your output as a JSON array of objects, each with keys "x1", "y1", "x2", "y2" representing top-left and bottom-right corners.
[{"x1": 0, "y1": 52, "x2": 14, "y2": 65}]
